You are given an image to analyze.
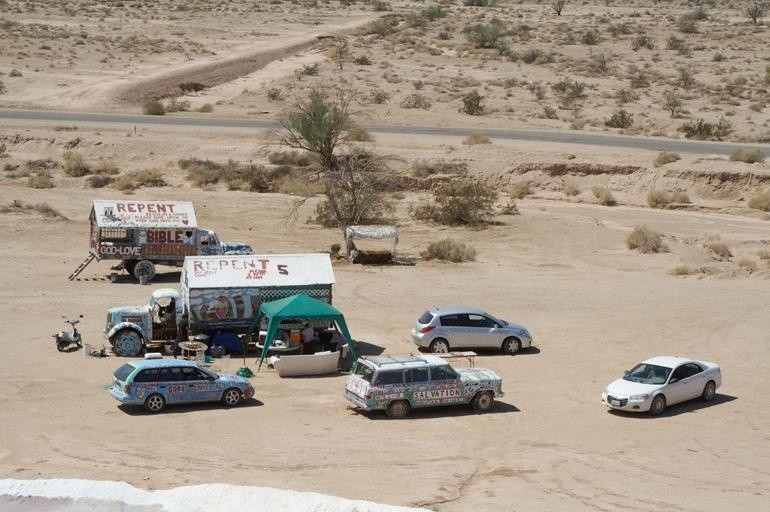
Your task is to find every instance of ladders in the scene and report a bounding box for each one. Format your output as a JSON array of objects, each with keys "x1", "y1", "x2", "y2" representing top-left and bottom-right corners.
[{"x1": 68, "y1": 252, "x2": 95, "y2": 281}]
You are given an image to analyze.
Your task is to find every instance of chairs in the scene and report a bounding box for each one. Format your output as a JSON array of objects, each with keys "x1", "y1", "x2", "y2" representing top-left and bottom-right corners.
[{"x1": 237, "y1": 334, "x2": 256, "y2": 366}]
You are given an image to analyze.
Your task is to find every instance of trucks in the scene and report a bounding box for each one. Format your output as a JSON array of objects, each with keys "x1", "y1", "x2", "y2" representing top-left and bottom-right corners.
[
  {"x1": 88, "y1": 200, "x2": 253, "y2": 282},
  {"x1": 103, "y1": 253, "x2": 336, "y2": 357}
]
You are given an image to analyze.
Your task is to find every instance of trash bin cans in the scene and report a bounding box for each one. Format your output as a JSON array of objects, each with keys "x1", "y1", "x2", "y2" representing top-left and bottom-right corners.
[
  {"x1": 140, "y1": 275, "x2": 147, "y2": 285},
  {"x1": 111, "y1": 273, "x2": 117, "y2": 283},
  {"x1": 83, "y1": 344, "x2": 91, "y2": 356},
  {"x1": 194, "y1": 334, "x2": 210, "y2": 357}
]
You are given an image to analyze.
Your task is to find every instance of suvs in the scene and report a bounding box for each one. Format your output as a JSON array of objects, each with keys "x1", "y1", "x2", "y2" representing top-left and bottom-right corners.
[{"x1": 343, "y1": 353, "x2": 504, "y2": 418}]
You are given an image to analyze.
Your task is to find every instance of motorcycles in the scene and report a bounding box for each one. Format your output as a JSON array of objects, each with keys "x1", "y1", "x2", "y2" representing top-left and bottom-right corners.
[{"x1": 52, "y1": 314, "x2": 83, "y2": 352}]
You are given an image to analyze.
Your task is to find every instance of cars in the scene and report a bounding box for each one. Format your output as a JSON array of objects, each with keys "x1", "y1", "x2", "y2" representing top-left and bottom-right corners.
[
  {"x1": 108, "y1": 353, "x2": 254, "y2": 413},
  {"x1": 601, "y1": 356, "x2": 721, "y2": 416},
  {"x1": 410, "y1": 307, "x2": 532, "y2": 353}
]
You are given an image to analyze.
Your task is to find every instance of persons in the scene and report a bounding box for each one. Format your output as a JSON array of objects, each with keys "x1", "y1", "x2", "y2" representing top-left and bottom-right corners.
[
  {"x1": 302, "y1": 323, "x2": 314, "y2": 355},
  {"x1": 318, "y1": 325, "x2": 333, "y2": 345}
]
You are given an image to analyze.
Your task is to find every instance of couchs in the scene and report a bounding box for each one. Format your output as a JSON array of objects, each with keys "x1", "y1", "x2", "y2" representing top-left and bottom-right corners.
[{"x1": 270, "y1": 351, "x2": 341, "y2": 377}]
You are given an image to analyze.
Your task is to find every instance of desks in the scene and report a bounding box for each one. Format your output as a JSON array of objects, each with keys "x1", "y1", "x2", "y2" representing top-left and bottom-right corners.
[
  {"x1": 432, "y1": 350, "x2": 478, "y2": 368},
  {"x1": 178, "y1": 340, "x2": 208, "y2": 360},
  {"x1": 256, "y1": 340, "x2": 304, "y2": 364}
]
